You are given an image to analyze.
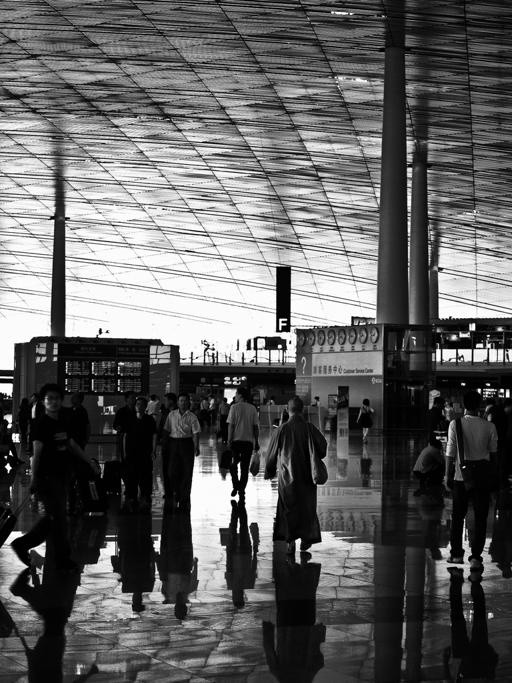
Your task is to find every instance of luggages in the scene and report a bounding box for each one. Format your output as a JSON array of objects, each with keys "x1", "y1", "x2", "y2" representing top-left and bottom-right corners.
[
  {"x1": 0, "y1": 496, "x2": 31, "y2": 548},
  {"x1": 81, "y1": 460, "x2": 121, "y2": 514}
]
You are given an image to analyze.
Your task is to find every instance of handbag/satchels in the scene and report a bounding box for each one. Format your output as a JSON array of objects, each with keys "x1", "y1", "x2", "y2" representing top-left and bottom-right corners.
[
  {"x1": 250, "y1": 453, "x2": 260, "y2": 476},
  {"x1": 460, "y1": 459, "x2": 491, "y2": 490},
  {"x1": 310, "y1": 451, "x2": 328, "y2": 484}
]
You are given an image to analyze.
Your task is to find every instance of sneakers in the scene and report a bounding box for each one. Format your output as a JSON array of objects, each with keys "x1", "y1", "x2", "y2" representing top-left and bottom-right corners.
[{"x1": 447, "y1": 558, "x2": 481, "y2": 568}]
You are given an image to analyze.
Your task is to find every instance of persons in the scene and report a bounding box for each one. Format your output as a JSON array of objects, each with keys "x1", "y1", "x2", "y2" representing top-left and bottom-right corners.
[{"x1": 1, "y1": 383, "x2": 511, "y2": 574}]
